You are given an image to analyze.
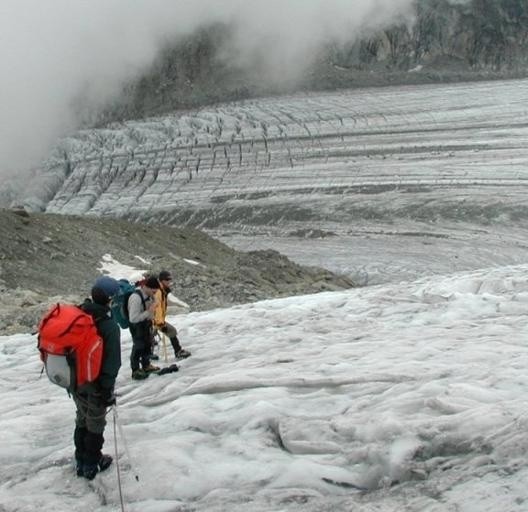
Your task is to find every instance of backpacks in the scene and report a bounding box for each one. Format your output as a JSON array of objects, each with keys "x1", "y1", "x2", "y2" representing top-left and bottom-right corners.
[
  {"x1": 38, "y1": 303, "x2": 103, "y2": 393},
  {"x1": 111, "y1": 280, "x2": 145, "y2": 328}
]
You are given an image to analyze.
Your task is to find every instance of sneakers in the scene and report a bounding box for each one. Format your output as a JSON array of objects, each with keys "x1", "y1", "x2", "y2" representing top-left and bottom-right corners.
[
  {"x1": 77, "y1": 455, "x2": 111, "y2": 478},
  {"x1": 132, "y1": 354, "x2": 160, "y2": 379},
  {"x1": 176, "y1": 350, "x2": 190, "y2": 357}
]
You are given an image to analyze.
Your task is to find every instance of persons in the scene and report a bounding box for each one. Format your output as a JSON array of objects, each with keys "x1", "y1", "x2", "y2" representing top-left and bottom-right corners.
[
  {"x1": 73, "y1": 276, "x2": 121, "y2": 479},
  {"x1": 149, "y1": 270, "x2": 191, "y2": 360},
  {"x1": 127, "y1": 277, "x2": 160, "y2": 380}
]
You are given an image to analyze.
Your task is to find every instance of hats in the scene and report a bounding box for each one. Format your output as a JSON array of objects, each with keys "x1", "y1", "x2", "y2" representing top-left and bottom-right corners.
[
  {"x1": 146, "y1": 277, "x2": 161, "y2": 289},
  {"x1": 92, "y1": 277, "x2": 119, "y2": 297},
  {"x1": 159, "y1": 271, "x2": 171, "y2": 280}
]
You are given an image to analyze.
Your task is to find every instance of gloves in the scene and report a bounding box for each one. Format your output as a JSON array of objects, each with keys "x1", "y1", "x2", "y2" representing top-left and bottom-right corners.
[{"x1": 102, "y1": 387, "x2": 115, "y2": 407}]
